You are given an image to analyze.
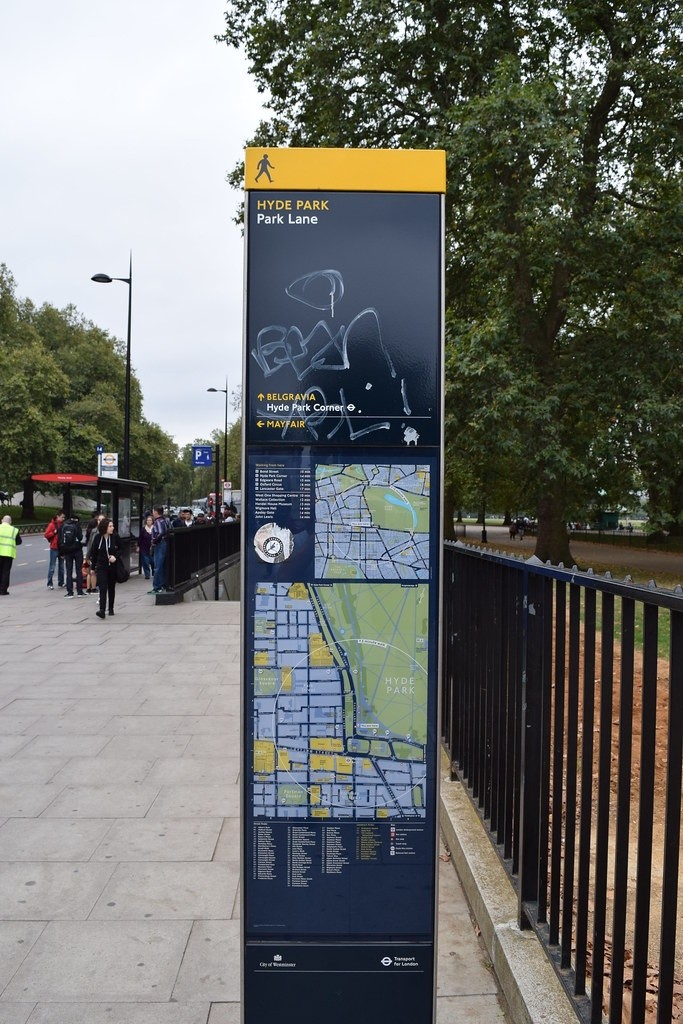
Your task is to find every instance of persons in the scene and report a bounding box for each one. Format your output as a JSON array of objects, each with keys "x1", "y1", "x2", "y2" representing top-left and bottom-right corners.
[
  {"x1": 0, "y1": 515, "x2": 22, "y2": 595},
  {"x1": 84, "y1": 515, "x2": 108, "y2": 591},
  {"x1": 85, "y1": 511, "x2": 99, "y2": 546},
  {"x1": 139, "y1": 495, "x2": 239, "y2": 592},
  {"x1": 506, "y1": 512, "x2": 634, "y2": 539},
  {"x1": 58, "y1": 514, "x2": 85, "y2": 598},
  {"x1": 44, "y1": 509, "x2": 70, "y2": 591},
  {"x1": 88, "y1": 516, "x2": 123, "y2": 619}
]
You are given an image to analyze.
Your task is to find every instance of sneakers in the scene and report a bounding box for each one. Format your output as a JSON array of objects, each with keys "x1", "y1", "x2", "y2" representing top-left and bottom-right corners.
[
  {"x1": 47, "y1": 585, "x2": 54, "y2": 590},
  {"x1": 147, "y1": 588, "x2": 163, "y2": 595},
  {"x1": 77, "y1": 592, "x2": 88, "y2": 598},
  {"x1": 64, "y1": 592, "x2": 75, "y2": 598},
  {"x1": 58, "y1": 584, "x2": 67, "y2": 589}
]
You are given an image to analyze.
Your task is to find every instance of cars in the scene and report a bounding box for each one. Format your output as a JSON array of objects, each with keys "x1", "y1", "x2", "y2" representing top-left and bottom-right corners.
[{"x1": 162, "y1": 504, "x2": 203, "y2": 519}]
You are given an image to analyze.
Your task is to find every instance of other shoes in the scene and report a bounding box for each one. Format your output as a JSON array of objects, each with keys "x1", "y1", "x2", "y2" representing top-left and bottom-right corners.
[
  {"x1": 86, "y1": 588, "x2": 91, "y2": 595},
  {"x1": 109, "y1": 608, "x2": 113, "y2": 615},
  {"x1": 145, "y1": 576, "x2": 149, "y2": 579},
  {"x1": 91, "y1": 589, "x2": 98, "y2": 593},
  {"x1": 96, "y1": 609, "x2": 105, "y2": 618},
  {"x1": 0, "y1": 592, "x2": 9, "y2": 595}
]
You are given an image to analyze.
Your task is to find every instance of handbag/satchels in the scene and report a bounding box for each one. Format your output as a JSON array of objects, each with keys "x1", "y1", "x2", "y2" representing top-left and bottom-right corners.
[{"x1": 115, "y1": 553, "x2": 129, "y2": 584}]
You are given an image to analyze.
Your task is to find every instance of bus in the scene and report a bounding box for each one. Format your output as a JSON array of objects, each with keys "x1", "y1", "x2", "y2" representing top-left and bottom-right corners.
[
  {"x1": 192, "y1": 498, "x2": 207, "y2": 514},
  {"x1": 207, "y1": 493, "x2": 224, "y2": 518}
]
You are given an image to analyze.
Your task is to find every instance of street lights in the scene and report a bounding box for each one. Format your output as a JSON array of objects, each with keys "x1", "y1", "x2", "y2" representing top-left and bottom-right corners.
[
  {"x1": 207, "y1": 376, "x2": 228, "y2": 481},
  {"x1": 90, "y1": 247, "x2": 133, "y2": 481}
]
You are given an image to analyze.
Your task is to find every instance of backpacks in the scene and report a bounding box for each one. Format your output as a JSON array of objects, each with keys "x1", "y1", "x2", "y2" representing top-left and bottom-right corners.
[{"x1": 59, "y1": 521, "x2": 80, "y2": 553}]
